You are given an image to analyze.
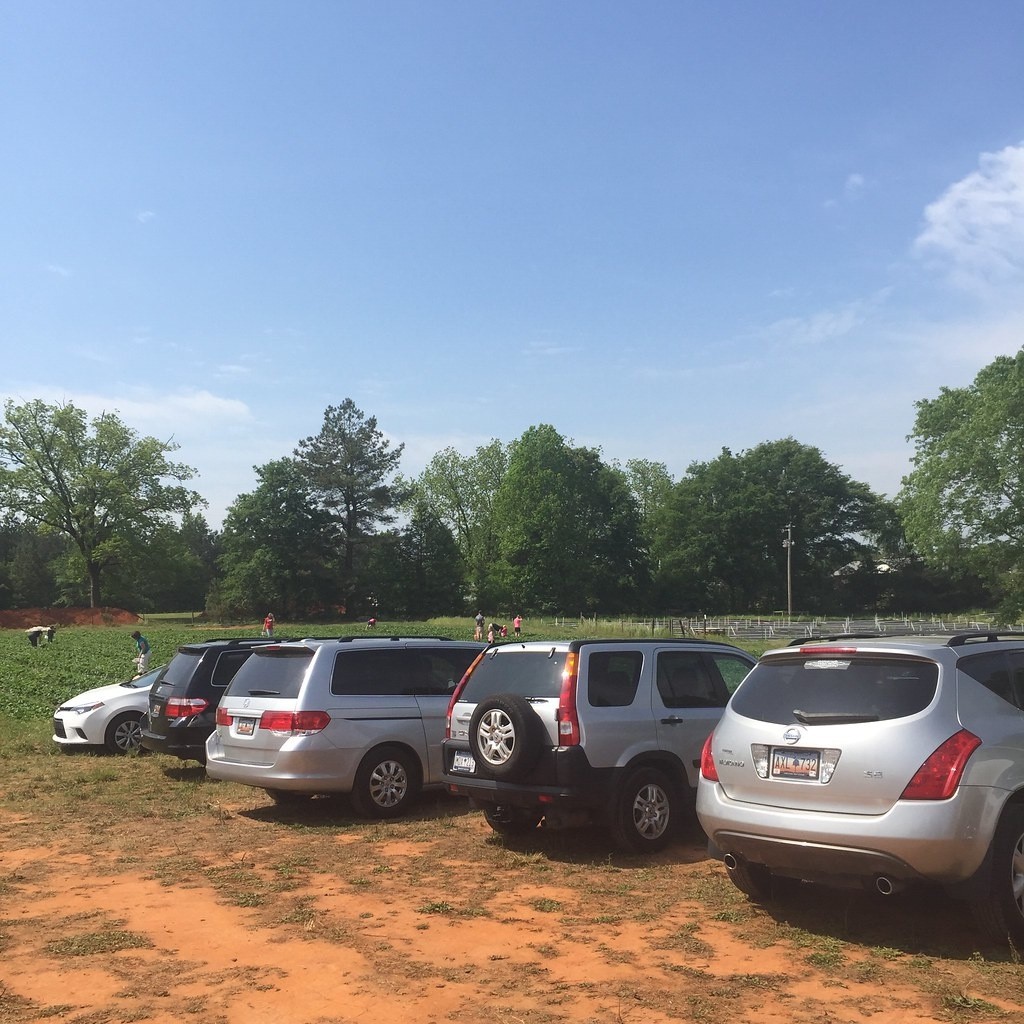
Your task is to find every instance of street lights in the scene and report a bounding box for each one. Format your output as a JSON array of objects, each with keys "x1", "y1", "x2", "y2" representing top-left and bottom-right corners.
[{"x1": 779, "y1": 521, "x2": 797, "y2": 630}]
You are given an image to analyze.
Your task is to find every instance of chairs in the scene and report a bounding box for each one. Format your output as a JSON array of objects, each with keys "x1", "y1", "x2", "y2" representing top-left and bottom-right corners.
[
  {"x1": 663, "y1": 666, "x2": 703, "y2": 703},
  {"x1": 605, "y1": 669, "x2": 633, "y2": 705}
]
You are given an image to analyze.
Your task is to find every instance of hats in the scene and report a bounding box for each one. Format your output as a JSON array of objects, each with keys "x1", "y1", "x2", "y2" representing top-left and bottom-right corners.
[
  {"x1": 131, "y1": 630, "x2": 141, "y2": 639},
  {"x1": 479, "y1": 610, "x2": 483, "y2": 613}
]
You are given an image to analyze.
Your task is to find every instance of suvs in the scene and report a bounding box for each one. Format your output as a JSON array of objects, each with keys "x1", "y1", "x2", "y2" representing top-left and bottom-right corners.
[
  {"x1": 140, "y1": 637, "x2": 308, "y2": 768},
  {"x1": 201, "y1": 634, "x2": 501, "y2": 823},
  {"x1": 694, "y1": 629, "x2": 1023, "y2": 952},
  {"x1": 438, "y1": 637, "x2": 784, "y2": 857}
]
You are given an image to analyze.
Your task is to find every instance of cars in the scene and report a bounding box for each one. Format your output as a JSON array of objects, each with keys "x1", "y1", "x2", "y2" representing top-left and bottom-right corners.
[{"x1": 52, "y1": 662, "x2": 174, "y2": 757}]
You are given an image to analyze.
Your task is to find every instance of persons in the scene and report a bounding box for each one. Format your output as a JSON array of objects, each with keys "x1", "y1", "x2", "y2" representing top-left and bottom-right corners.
[
  {"x1": 263, "y1": 612, "x2": 275, "y2": 637},
  {"x1": 47, "y1": 626, "x2": 57, "y2": 644},
  {"x1": 513, "y1": 614, "x2": 522, "y2": 638},
  {"x1": 366, "y1": 618, "x2": 377, "y2": 630},
  {"x1": 475, "y1": 610, "x2": 485, "y2": 642},
  {"x1": 487, "y1": 622, "x2": 508, "y2": 642},
  {"x1": 131, "y1": 631, "x2": 151, "y2": 674},
  {"x1": 28, "y1": 631, "x2": 43, "y2": 648}
]
